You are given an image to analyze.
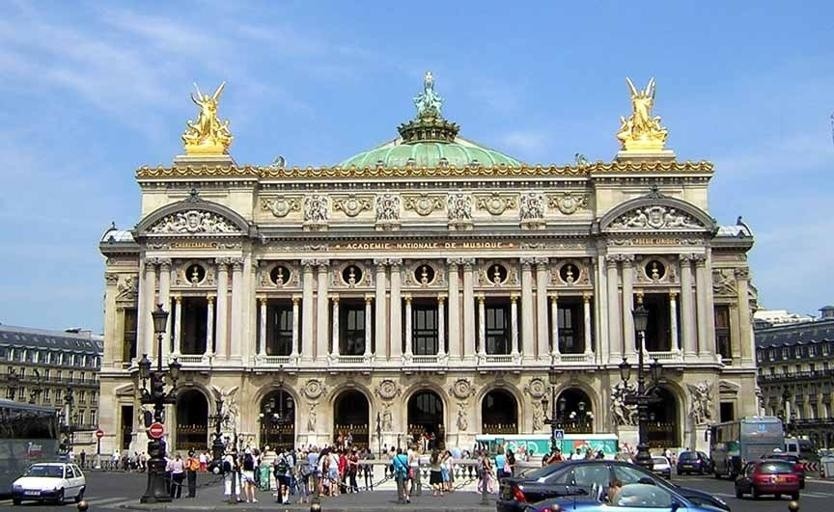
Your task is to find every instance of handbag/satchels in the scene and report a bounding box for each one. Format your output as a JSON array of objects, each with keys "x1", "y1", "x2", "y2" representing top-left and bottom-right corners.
[
  {"x1": 323, "y1": 457, "x2": 329, "y2": 473},
  {"x1": 407, "y1": 469, "x2": 409, "y2": 479},
  {"x1": 504, "y1": 455, "x2": 512, "y2": 473},
  {"x1": 182, "y1": 463, "x2": 186, "y2": 479}
]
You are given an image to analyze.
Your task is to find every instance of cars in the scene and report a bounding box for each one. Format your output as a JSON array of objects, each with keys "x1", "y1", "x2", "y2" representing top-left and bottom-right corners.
[
  {"x1": 206, "y1": 458, "x2": 224, "y2": 475},
  {"x1": 761, "y1": 452, "x2": 805, "y2": 489},
  {"x1": 677, "y1": 452, "x2": 711, "y2": 475},
  {"x1": 652, "y1": 456, "x2": 671, "y2": 479},
  {"x1": 13, "y1": 462, "x2": 86, "y2": 505},
  {"x1": 734, "y1": 458, "x2": 799, "y2": 498},
  {"x1": 497, "y1": 459, "x2": 730, "y2": 512}
]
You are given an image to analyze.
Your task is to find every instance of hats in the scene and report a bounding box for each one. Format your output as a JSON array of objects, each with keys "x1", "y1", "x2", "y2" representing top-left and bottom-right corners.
[
  {"x1": 188, "y1": 450, "x2": 195, "y2": 457},
  {"x1": 550, "y1": 446, "x2": 559, "y2": 450}
]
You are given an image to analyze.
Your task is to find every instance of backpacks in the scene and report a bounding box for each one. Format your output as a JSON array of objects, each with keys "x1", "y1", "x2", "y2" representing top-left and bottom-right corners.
[
  {"x1": 188, "y1": 458, "x2": 199, "y2": 472},
  {"x1": 244, "y1": 453, "x2": 253, "y2": 470},
  {"x1": 224, "y1": 461, "x2": 231, "y2": 471},
  {"x1": 278, "y1": 463, "x2": 287, "y2": 473}
]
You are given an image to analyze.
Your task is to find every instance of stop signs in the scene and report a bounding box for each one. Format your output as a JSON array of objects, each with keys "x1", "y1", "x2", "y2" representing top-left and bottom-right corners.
[
  {"x1": 96, "y1": 430, "x2": 103, "y2": 438},
  {"x1": 149, "y1": 424, "x2": 165, "y2": 438}
]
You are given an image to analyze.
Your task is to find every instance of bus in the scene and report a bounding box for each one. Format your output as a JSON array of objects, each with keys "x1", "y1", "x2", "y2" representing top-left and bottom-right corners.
[
  {"x1": 473, "y1": 434, "x2": 618, "y2": 460},
  {"x1": 705, "y1": 417, "x2": 785, "y2": 478},
  {"x1": 0, "y1": 398, "x2": 59, "y2": 494}
]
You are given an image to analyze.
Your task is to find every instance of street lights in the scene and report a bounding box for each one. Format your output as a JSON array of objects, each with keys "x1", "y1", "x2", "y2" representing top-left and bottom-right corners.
[
  {"x1": 618, "y1": 306, "x2": 662, "y2": 470},
  {"x1": 570, "y1": 402, "x2": 595, "y2": 434},
  {"x1": 138, "y1": 304, "x2": 181, "y2": 503},
  {"x1": 541, "y1": 366, "x2": 567, "y2": 454},
  {"x1": 209, "y1": 400, "x2": 229, "y2": 459},
  {"x1": 257, "y1": 370, "x2": 294, "y2": 446}
]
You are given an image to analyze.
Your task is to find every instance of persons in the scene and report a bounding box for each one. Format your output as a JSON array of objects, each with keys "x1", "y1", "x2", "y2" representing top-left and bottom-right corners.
[
  {"x1": 382, "y1": 432, "x2": 519, "y2": 505},
  {"x1": 191, "y1": 93, "x2": 217, "y2": 136},
  {"x1": 165, "y1": 443, "x2": 376, "y2": 506},
  {"x1": 70, "y1": 447, "x2": 147, "y2": 472},
  {"x1": 631, "y1": 90, "x2": 653, "y2": 136},
  {"x1": 541, "y1": 442, "x2": 678, "y2": 506}
]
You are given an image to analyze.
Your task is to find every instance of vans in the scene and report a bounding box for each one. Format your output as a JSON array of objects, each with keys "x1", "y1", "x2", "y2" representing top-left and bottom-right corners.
[{"x1": 784, "y1": 439, "x2": 814, "y2": 456}]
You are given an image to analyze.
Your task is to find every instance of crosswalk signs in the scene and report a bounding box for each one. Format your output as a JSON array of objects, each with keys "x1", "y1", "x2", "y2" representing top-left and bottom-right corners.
[{"x1": 554, "y1": 429, "x2": 564, "y2": 440}]
